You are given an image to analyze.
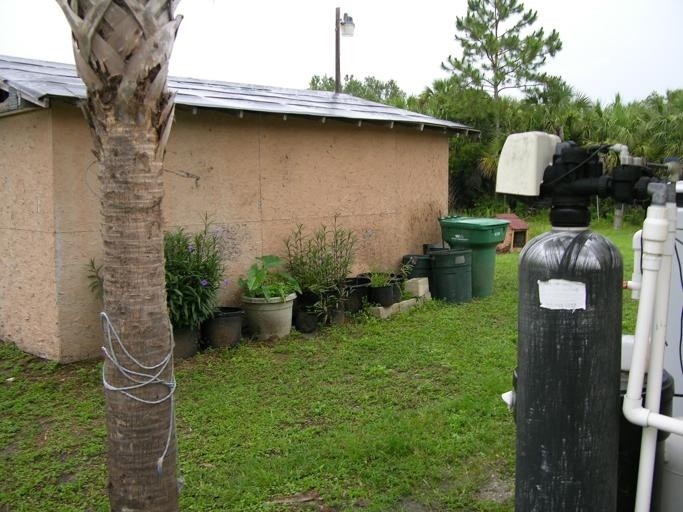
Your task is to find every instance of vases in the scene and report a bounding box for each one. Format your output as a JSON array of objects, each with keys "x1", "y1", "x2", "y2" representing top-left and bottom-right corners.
[
  {"x1": 167, "y1": 319, "x2": 201, "y2": 360},
  {"x1": 206, "y1": 305, "x2": 245, "y2": 349},
  {"x1": 335, "y1": 271, "x2": 406, "y2": 312}
]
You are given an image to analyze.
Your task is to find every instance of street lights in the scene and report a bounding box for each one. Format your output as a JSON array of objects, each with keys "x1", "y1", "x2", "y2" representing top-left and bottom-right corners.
[{"x1": 334, "y1": 7, "x2": 355, "y2": 93}]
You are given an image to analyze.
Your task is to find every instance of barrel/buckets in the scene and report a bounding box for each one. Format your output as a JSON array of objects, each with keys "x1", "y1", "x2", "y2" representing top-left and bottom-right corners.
[{"x1": 428, "y1": 248, "x2": 474, "y2": 302}]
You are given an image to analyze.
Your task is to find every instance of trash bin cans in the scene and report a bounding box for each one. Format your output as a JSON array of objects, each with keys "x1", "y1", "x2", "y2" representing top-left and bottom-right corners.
[{"x1": 428, "y1": 215, "x2": 509, "y2": 302}]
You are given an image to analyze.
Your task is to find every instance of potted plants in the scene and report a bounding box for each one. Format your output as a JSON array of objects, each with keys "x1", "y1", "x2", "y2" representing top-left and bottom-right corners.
[{"x1": 237, "y1": 211, "x2": 354, "y2": 341}]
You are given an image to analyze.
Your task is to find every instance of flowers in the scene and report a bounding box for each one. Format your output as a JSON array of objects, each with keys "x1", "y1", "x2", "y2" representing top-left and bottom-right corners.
[{"x1": 99, "y1": 216, "x2": 229, "y2": 324}]
